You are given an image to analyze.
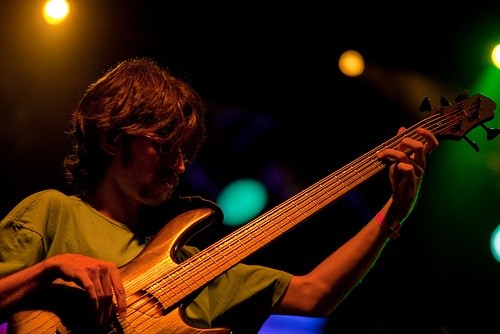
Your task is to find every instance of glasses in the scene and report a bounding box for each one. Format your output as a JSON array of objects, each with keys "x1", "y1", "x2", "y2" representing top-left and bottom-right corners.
[{"x1": 144, "y1": 133, "x2": 185, "y2": 154}]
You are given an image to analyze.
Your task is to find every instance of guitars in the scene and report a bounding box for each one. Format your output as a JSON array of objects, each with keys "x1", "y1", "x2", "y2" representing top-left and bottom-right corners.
[{"x1": 0, "y1": 86, "x2": 500, "y2": 334}]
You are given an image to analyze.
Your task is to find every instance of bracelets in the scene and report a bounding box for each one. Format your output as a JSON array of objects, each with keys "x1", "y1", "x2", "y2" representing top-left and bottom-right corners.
[{"x1": 376, "y1": 212, "x2": 401, "y2": 241}]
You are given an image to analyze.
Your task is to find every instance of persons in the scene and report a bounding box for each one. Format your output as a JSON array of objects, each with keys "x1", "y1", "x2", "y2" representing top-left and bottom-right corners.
[{"x1": 0, "y1": 55, "x2": 439, "y2": 334}]
